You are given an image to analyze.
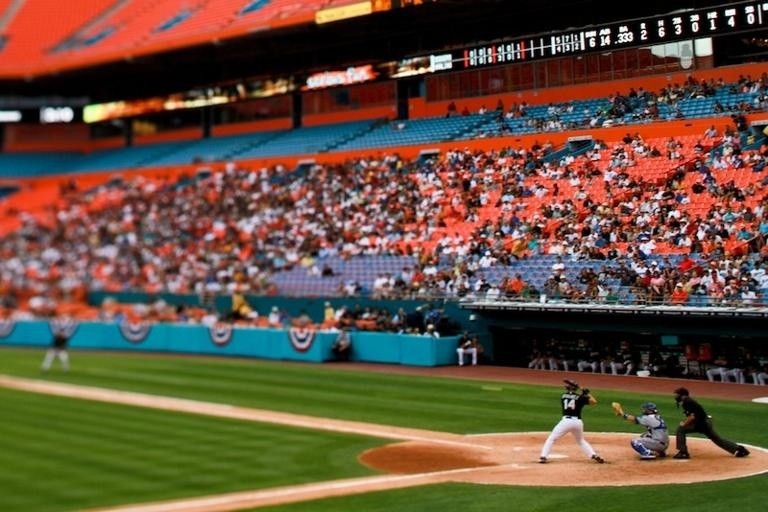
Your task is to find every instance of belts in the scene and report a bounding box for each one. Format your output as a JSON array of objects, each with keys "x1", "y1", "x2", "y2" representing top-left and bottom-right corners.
[
  {"x1": 660, "y1": 441, "x2": 665, "y2": 445},
  {"x1": 566, "y1": 417, "x2": 581, "y2": 419}
]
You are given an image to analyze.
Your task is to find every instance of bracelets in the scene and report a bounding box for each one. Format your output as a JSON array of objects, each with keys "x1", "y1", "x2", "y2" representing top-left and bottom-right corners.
[{"x1": 623, "y1": 413, "x2": 629, "y2": 419}]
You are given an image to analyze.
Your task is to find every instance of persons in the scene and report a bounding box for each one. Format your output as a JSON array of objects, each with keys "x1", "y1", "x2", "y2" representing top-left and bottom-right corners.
[
  {"x1": 537, "y1": 379, "x2": 604, "y2": 463},
  {"x1": 611, "y1": 400, "x2": 670, "y2": 460},
  {"x1": 446, "y1": 139, "x2": 553, "y2": 154},
  {"x1": 42, "y1": 319, "x2": 78, "y2": 372},
  {"x1": 672, "y1": 387, "x2": 750, "y2": 458},
  {"x1": 528, "y1": 335, "x2": 768, "y2": 387},
  {"x1": 1, "y1": 157, "x2": 768, "y2": 308},
  {"x1": 332, "y1": 330, "x2": 353, "y2": 361},
  {"x1": 456, "y1": 331, "x2": 482, "y2": 368},
  {"x1": 581, "y1": 71, "x2": 768, "y2": 155},
  {"x1": 0, "y1": 290, "x2": 459, "y2": 338},
  {"x1": 445, "y1": 99, "x2": 581, "y2": 141}
]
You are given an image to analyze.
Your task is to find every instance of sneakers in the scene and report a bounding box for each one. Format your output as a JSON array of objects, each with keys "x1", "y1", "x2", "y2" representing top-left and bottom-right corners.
[
  {"x1": 672, "y1": 451, "x2": 688, "y2": 458},
  {"x1": 736, "y1": 449, "x2": 749, "y2": 457},
  {"x1": 656, "y1": 451, "x2": 666, "y2": 457},
  {"x1": 539, "y1": 457, "x2": 546, "y2": 462},
  {"x1": 595, "y1": 455, "x2": 604, "y2": 463},
  {"x1": 640, "y1": 454, "x2": 656, "y2": 459}
]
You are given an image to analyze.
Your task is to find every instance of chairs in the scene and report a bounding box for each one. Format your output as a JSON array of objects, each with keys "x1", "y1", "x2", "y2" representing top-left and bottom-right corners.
[{"x1": 0, "y1": 81, "x2": 768, "y2": 305}]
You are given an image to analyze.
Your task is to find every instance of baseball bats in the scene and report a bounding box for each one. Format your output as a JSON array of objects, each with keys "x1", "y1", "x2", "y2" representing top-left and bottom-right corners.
[{"x1": 564, "y1": 379, "x2": 587, "y2": 392}]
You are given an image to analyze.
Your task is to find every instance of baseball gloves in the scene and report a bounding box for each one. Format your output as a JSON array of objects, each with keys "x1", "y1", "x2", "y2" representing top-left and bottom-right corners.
[{"x1": 612, "y1": 402, "x2": 623, "y2": 416}]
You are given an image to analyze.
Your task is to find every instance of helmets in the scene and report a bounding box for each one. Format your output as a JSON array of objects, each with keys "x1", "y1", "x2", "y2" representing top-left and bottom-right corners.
[
  {"x1": 674, "y1": 387, "x2": 689, "y2": 402},
  {"x1": 642, "y1": 403, "x2": 658, "y2": 415},
  {"x1": 566, "y1": 380, "x2": 578, "y2": 390}
]
